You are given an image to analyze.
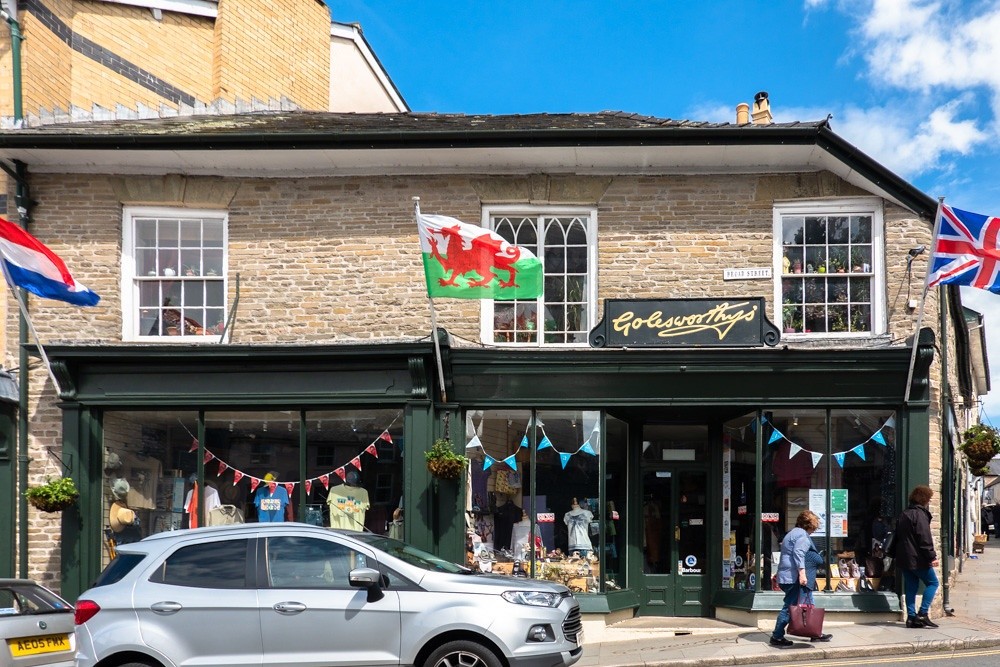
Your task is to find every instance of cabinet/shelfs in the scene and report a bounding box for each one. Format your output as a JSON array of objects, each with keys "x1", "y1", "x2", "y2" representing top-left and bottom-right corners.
[{"x1": 148, "y1": 475, "x2": 194, "y2": 536}]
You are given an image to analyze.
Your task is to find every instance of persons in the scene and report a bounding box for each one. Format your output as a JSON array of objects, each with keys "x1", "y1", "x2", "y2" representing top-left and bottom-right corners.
[
  {"x1": 770, "y1": 509, "x2": 834, "y2": 646},
  {"x1": 895, "y1": 483, "x2": 941, "y2": 627}
]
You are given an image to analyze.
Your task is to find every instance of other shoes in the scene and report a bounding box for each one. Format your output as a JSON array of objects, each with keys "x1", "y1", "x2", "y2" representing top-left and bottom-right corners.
[
  {"x1": 771, "y1": 550, "x2": 898, "y2": 592},
  {"x1": 477, "y1": 545, "x2": 621, "y2": 594}
]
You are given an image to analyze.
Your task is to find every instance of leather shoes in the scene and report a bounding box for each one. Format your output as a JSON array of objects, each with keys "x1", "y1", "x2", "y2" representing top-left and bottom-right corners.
[
  {"x1": 906, "y1": 615, "x2": 927, "y2": 628},
  {"x1": 915, "y1": 612, "x2": 939, "y2": 627}
]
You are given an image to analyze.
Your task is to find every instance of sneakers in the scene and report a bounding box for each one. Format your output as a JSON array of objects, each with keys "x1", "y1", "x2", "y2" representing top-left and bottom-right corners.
[
  {"x1": 810, "y1": 631, "x2": 833, "y2": 642},
  {"x1": 769, "y1": 636, "x2": 794, "y2": 649}
]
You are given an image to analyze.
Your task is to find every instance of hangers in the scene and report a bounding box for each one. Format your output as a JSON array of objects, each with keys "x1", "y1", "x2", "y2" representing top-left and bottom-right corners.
[{"x1": 464, "y1": 441, "x2": 523, "y2": 511}]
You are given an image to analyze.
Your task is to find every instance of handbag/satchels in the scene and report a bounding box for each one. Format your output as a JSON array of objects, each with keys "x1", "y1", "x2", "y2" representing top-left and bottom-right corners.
[
  {"x1": 495, "y1": 468, "x2": 518, "y2": 496},
  {"x1": 786, "y1": 584, "x2": 825, "y2": 639},
  {"x1": 882, "y1": 532, "x2": 898, "y2": 558}
]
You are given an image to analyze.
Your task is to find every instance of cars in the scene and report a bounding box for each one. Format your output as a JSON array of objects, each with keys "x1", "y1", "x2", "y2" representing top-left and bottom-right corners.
[{"x1": 1, "y1": 579, "x2": 78, "y2": 667}]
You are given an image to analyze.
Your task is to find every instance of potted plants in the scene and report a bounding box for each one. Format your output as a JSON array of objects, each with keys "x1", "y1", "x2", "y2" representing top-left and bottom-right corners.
[
  {"x1": 782, "y1": 240, "x2": 872, "y2": 275},
  {"x1": 147, "y1": 262, "x2": 219, "y2": 277},
  {"x1": 424, "y1": 437, "x2": 470, "y2": 493},
  {"x1": 166, "y1": 314, "x2": 224, "y2": 336},
  {"x1": 20, "y1": 473, "x2": 79, "y2": 513},
  {"x1": 782, "y1": 281, "x2": 870, "y2": 331},
  {"x1": 957, "y1": 418, "x2": 1000, "y2": 476}
]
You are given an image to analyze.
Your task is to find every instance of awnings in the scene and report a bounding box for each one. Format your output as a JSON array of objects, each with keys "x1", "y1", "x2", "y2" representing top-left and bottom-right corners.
[{"x1": 984, "y1": 474, "x2": 1000, "y2": 489}]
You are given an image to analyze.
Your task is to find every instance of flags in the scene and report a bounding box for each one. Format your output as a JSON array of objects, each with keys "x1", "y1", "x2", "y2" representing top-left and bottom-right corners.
[
  {"x1": 928, "y1": 203, "x2": 1000, "y2": 295},
  {"x1": 0, "y1": 217, "x2": 101, "y2": 309},
  {"x1": 416, "y1": 212, "x2": 543, "y2": 298}
]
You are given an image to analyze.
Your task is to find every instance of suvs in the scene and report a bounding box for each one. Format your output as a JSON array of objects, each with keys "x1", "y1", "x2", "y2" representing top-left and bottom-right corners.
[{"x1": 72, "y1": 521, "x2": 584, "y2": 667}]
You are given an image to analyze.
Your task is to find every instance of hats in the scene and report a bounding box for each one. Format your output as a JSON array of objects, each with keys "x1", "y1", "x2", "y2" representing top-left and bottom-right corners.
[{"x1": 104, "y1": 450, "x2": 135, "y2": 532}]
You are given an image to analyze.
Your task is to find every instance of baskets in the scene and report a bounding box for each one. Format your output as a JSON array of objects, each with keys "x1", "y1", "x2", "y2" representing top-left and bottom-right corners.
[
  {"x1": 964, "y1": 428, "x2": 998, "y2": 477},
  {"x1": 975, "y1": 531, "x2": 988, "y2": 543},
  {"x1": 29, "y1": 494, "x2": 73, "y2": 513},
  {"x1": 428, "y1": 458, "x2": 462, "y2": 479}
]
